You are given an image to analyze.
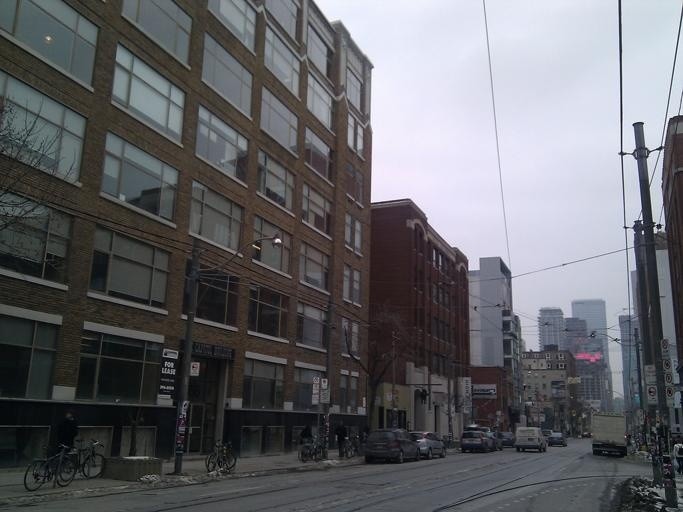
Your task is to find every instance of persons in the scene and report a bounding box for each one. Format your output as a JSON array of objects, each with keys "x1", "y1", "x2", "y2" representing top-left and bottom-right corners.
[
  {"x1": 53, "y1": 410, "x2": 80, "y2": 453},
  {"x1": 300, "y1": 425, "x2": 317, "y2": 457},
  {"x1": 334, "y1": 420, "x2": 347, "y2": 456}
]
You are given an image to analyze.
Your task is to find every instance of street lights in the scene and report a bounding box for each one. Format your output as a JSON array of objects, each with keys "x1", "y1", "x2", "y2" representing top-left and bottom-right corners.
[
  {"x1": 589, "y1": 329, "x2": 650, "y2": 439},
  {"x1": 173, "y1": 232, "x2": 281, "y2": 473}
]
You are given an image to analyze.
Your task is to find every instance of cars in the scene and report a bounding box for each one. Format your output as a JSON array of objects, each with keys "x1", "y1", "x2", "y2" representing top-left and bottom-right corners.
[
  {"x1": 460, "y1": 423, "x2": 515, "y2": 452},
  {"x1": 408, "y1": 431, "x2": 445, "y2": 460},
  {"x1": 542, "y1": 430, "x2": 567, "y2": 447}
]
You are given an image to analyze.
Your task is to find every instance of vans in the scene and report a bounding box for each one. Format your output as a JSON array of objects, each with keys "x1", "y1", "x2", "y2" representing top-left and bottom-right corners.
[{"x1": 517, "y1": 427, "x2": 547, "y2": 453}]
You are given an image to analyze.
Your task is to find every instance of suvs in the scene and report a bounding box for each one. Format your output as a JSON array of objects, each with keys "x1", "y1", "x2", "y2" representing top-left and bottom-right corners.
[{"x1": 366, "y1": 429, "x2": 420, "y2": 463}]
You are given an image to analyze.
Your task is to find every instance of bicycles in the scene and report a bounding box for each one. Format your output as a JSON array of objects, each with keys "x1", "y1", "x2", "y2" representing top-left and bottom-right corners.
[
  {"x1": 205, "y1": 439, "x2": 237, "y2": 473},
  {"x1": 344, "y1": 432, "x2": 360, "y2": 459},
  {"x1": 299, "y1": 435, "x2": 327, "y2": 464},
  {"x1": 23, "y1": 437, "x2": 105, "y2": 491}
]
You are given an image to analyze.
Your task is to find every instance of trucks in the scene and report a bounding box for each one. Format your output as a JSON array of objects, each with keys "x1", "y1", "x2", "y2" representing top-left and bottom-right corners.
[{"x1": 592, "y1": 412, "x2": 628, "y2": 458}]
